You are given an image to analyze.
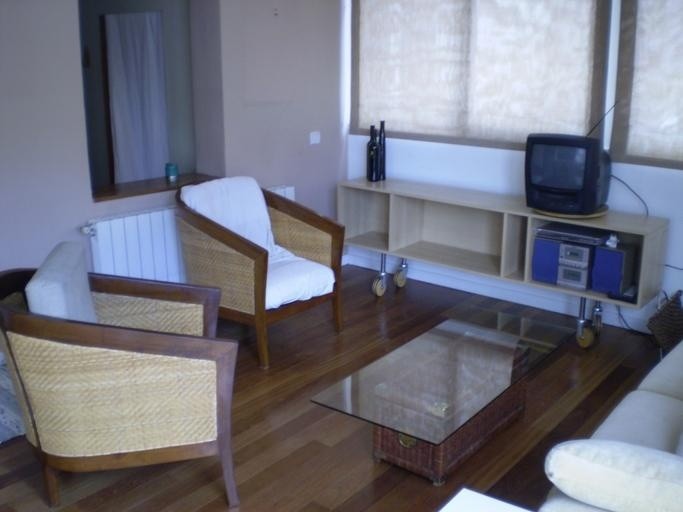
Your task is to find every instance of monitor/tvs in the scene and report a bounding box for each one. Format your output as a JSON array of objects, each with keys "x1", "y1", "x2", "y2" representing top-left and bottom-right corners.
[{"x1": 526, "y1": 134, "x2": 611, "y2": 215}]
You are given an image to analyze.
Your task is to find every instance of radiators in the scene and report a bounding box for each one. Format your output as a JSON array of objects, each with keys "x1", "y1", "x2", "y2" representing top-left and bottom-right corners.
[{"x1": 80, "y1": 186, "x2": 295, "y2": 286}]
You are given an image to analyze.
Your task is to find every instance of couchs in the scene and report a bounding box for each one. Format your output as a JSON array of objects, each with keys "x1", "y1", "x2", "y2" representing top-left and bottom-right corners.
[{"x1": 439, "y1": 291, "x2": 683, "y2": 512}]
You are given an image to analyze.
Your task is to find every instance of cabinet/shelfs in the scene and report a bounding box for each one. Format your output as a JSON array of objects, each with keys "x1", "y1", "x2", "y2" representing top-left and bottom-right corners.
[{"x1": 336, "y1": 173, "x2": 669, "y2": 349}]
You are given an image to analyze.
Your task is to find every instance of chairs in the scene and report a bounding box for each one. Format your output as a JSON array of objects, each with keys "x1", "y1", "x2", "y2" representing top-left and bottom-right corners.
[
  {"x1": 0, "y1": 266, "x2": 239, "y2": 511},
  {"x1": 173, "y1": 175, "x2": 346, "y2": 371}
]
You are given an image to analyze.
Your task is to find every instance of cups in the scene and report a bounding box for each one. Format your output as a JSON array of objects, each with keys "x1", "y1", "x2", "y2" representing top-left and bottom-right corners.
[{"x1": 165, "y1": 162, "x2": 179, "y2": 184}]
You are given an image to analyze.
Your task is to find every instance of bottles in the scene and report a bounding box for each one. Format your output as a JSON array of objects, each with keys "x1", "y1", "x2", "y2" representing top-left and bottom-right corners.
[{"x1": 366, "y1": 120, "x2": 386, "y2": 183}]
